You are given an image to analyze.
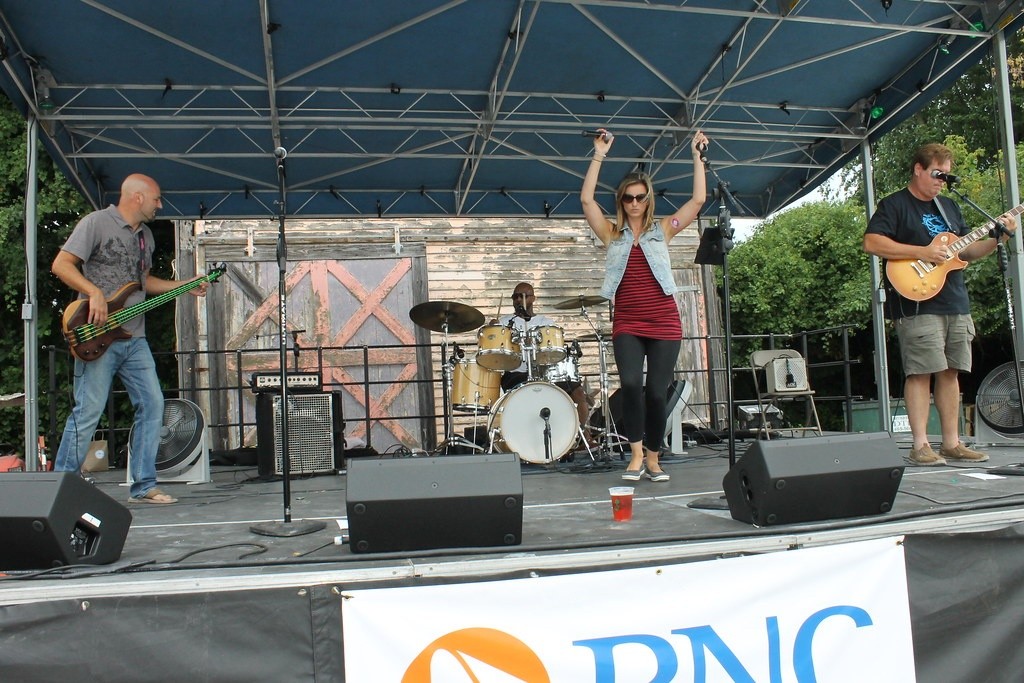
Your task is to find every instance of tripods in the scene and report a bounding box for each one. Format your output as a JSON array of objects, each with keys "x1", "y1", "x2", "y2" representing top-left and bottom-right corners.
[
  {"x1": 581, "y1": 307, "x2": 629, "y2": 465},
  {"x1": 430, "y1": 322, "x2": 487, "y2": 456}
]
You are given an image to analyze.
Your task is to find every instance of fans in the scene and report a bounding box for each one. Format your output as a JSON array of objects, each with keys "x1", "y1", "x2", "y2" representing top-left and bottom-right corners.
[
  {"x1": 118, "y1": 398, "x2": 210, "y2": 487},
  {"x1": 973, "y1": 360, "x2": 1024, "y2": 447}
]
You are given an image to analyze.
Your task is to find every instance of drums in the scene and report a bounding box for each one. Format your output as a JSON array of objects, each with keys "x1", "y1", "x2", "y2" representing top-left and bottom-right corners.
[
  {"x1": 488, "y1": 381, "x2": 581, "y2": 467},
  {"x1": 448, "y1": 359, "x2": 503, "y2": 416},
  {"x1": 525, "y1": 324, "x2": 568, "y2": 366},
  {"x1": 475, "y1": 324, "x2": 523, "y2": 371}
]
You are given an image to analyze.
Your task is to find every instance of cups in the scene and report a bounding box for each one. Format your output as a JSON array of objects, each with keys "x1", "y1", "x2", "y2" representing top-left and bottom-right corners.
[{"x1": 609, "y1": 487, "x2": 635, "y2": 523}]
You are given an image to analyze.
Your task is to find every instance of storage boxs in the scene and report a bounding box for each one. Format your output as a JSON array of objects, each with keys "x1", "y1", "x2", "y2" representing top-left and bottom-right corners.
[{"x1": 738, "y1": 405, "x2": 784, "y2": 428}]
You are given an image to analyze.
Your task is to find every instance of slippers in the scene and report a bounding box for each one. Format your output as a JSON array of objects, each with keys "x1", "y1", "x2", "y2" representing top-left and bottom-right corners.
[{"x1": 128, "y1": 489, "x2": 178, "y2": 503}]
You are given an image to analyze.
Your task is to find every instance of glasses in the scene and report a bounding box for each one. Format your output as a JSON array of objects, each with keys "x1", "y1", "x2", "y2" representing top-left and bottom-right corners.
[
  {"x1": 928, "y1": 168, "x2": 951, "y2": 182},
  {"x1": 622, "y1": 193, "x2": 649, "y2": 205},
  {"x1": 511, "y1": 293, "x2": 533, "y2": 301}
]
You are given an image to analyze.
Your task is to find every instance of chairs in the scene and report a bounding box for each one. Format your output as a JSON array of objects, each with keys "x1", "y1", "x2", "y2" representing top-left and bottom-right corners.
[{"x1": 750, "y1": 349, "x2": 823, "y2": 440}]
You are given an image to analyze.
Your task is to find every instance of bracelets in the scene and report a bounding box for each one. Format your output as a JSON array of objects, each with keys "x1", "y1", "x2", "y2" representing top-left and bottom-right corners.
[{"x1": 591, "y1": 151, "x2": 607, "y2": 163}]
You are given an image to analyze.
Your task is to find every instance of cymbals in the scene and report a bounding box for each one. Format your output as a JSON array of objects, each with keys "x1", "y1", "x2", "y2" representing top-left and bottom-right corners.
[
  {"x1": 409, "y1": 301, "x2": 486, "y2": 335},
  {"x1": 553, "y1": 294, "x2": 611, "y2": 310},
  {"x1": 575, "y1": 332, "x2": 615, "y2": 344}
]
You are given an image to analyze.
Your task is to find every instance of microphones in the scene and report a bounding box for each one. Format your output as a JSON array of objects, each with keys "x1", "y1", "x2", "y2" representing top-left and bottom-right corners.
[
  {"x1": 452, "y1": 342, "x2": 465, "y2": 358},
  {"x1": 274, "y1": 147, "x2": 287, "y2": 159},
  {"x1": 520, "y1": 305, "x2": 531, "y2": 322},
  {"x1": 582, "y1": 130, "x2": 612, "y2": 140},
  {"x1": 931, "y1": 171, "x2": 961, "y2": 182},
  {"x1": 541, "y1": 408, "x2": 551, "y2": 436}
]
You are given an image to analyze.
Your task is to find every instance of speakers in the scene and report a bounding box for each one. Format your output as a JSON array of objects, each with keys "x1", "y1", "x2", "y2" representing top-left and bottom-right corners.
[
  {"x1": 345, "y1": 451, "x2": 524, "y2": 555},
  {"x1": 721, "y1": 429, "x2": 905, "y2": 527},
  {"x1": 0, "y1": 471, "x2": 133, "y2": 571},
  {"x1": 590, "y1": 386, "x2": 648, "y2": 452},
  {"x1": 255, "y1": 389, "x2": 345, "y2": 480}
]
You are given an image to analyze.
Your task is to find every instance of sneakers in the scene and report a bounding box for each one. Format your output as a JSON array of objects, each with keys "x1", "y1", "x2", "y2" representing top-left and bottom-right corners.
[
  {"x1": 909, "y1": 442, "x2": 946, "y2": 466},
  {"x1": 622, "y1": 461, "x2": 646, "y2": 481},
  {"x1": 643, "y1": 463, "x2": 670, "y2": 481},
  {"x1": 938, "y1": 440, "x2": 990, "y2": 462}
]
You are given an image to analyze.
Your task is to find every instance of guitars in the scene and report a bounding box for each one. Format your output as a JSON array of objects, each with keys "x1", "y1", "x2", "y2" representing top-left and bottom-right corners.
[
  {"x1": 62, "y1": 259, "x2": 228, "y2": 362},
  {"x1": 885, "y1": 202, "x2": 1024, "y2": 304}
]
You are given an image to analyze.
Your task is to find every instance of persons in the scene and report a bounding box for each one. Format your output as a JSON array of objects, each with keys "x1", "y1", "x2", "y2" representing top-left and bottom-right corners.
[
  {"x1": 581, "y1": 128, "x2": 709, "y2": 484},
  {"x1": 52, "y1": 173, "x2": 209, "y2": 505},
  {"x1": 864, "y1": 144, "x2": 1018, "y2": 467}
]
